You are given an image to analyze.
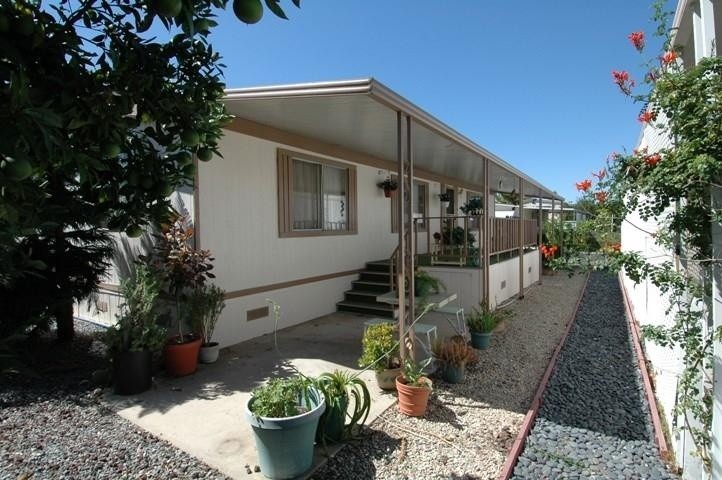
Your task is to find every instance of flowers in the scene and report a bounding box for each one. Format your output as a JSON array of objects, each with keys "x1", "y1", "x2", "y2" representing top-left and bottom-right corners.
[{"x1": 534, "y1": 0, "x2": 722, "y2": 308}]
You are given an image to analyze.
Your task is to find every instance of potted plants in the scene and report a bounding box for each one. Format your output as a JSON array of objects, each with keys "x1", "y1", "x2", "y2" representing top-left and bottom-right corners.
[
  {"x1": 245, "y1": 369, "x2": 372, "y2": 480},
  {"x1": 376, "y1": 178, "x2": 397, "y2": 197},
  {"x1": 413, "y1": 266, "x2": 447, "y2": 298},
  {"x1": 357, "y1": 306, "x2": 499, "y2": 417},
  {"x1": 102, "y1": 216, "x2": 227, "y2": 395}
]
